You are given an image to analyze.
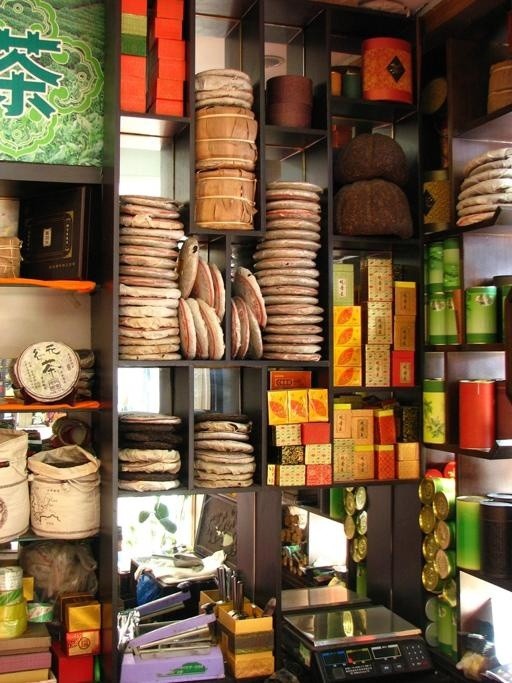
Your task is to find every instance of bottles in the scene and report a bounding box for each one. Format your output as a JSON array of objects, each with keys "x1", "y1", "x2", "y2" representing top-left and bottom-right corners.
[
  {"x1": 466, "y1": 286, "x2": 498, "y2": 343},
  {"x1": 425, "y1": 596, "x2": 439, "y2": 621},
  {"x1": 354, "y1": 487, "x2": 366, "y2": 511},
  {"x1": 485, "y1": 493, "x2": 512, "y2": 503},
  {"x1": 330, "y1": 487, "x2": 344, "y2": 518},
  {"x1": 437, "y1": 598, "x2": 452, "y2": 653},
  {"x1": 479, "y1": 501, "x2": 512, "y2": 580},
  {"x1": 434, "y1": 520, "x2": 457, "y2": 548},
  {"x1": 436, "y1": 550, "x2": 458, "y2": 578},
  {"x1": 443, "y1": 239, "x2": 461, "y2": 292},
  {"x1": 425, "y1": 623, "x2": 440, "y2": 648},
  {"x1": 428, "y1": 293, "x2": 446, "y2": 345},
  {"x1": 356, "y1": 511, "x2": 366, "y2": 535},
  {"x1": 423, "y1": 377, "x2": 445, "y2": 445},
  {"x1": 419, "y1": 507, "x2": 435, "y2": 533},
  {"x1": 423, "y1": 535, "x2": 438, "y2": 562},
  {"x1": 356, "y1": 563, "x2": 367, "y2": 596},
  {"x1": 343, "y1": 490, "x2": 354, "y2": 516},
  {"x1": 433, "y1": 492, "x2": 456, "y2": 522},
  {"x1": 451, "y1": 605, "x2": 459, "y2": 660},
  {"x1": 357, "y1": 536, "x2": 367, "y2": 560},
  {"x1": 444, "y1": 290, "x2": 464, "y2": 345},
  {"x1": 458, "y1": 379, "x2": 496, "y2": 452},
  {"x1": 421, "y1": 561, "x2": 446, "y2": 591},
  {"x1": 495, "y1": 380, "x2": 512, "y2": 441},
  {"x1": 427, "y1": 241, "x2": 443, "y2": 295},
  {"x1": 344, "y1": 516, "x2": 356, "y2": 540},
  {"x1": 456, "y1": 494, "x2": 490, "y2": 572},
  {"x1": 419, "y1": 477, "x2": 457, "y2": 504}
]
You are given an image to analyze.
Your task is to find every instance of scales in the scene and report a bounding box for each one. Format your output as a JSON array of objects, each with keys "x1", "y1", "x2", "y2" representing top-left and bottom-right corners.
[
  {"x1": 278, "y1": 586, "x2": 374, "y2": 614},
  {"x1": 278, "y1": 605, "x2": 433, "y2": 683}
]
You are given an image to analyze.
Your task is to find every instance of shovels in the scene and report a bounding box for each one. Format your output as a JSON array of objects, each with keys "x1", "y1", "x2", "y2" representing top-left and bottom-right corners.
[
  {"x1": 215, "y1": 563, "x2": 233, "y2": 603},
  {"x1": 227, "y1": 576, "x2": 277, "y2": 619},
  {"x1": 152, "y1": 554, "x2": 202, "y2": 568}
]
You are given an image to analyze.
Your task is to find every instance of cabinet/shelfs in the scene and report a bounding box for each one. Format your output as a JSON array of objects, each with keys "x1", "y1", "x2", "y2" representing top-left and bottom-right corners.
[{"x1": 0, "y1": 0, "x2": 511, "y2": 681}]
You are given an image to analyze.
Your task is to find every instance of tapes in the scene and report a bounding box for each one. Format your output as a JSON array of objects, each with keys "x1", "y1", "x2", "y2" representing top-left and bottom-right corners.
[
  {"x1": 28, "y1": 602, "x2": 54, "y2": 623},
  {"x1": 0, "y1": 565, "x2": 27, "y2": 639}
]
